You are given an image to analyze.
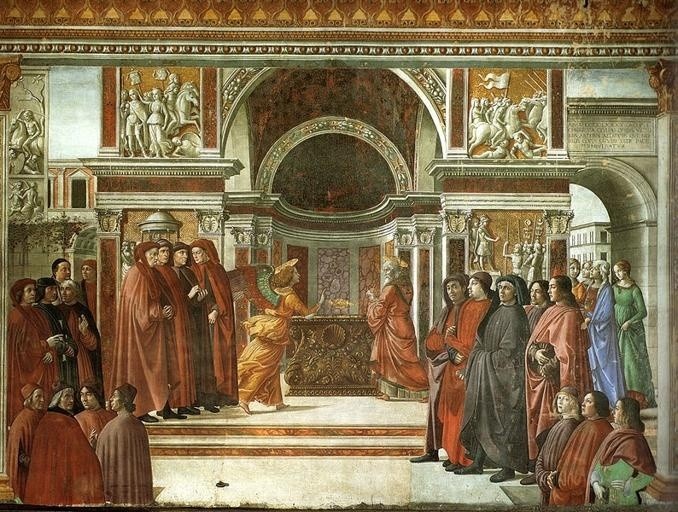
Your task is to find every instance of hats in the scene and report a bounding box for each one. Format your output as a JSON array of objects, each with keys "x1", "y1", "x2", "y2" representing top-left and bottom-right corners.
[
  {"x1": 559, "y1": 387, "x2": 578, "y2": 397},
  {"x1": 473, "y1": 272, "x2": 492, "y2": 287},
  {"x1": 21, "y1": 377, "x2": 137, "y2": 401}
]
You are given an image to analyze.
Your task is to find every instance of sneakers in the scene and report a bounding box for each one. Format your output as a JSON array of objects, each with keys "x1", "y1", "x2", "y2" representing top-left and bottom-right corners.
[
  {"x1": 239, "y1": 402, "x2": 251, "y2": 414},
  {"x1": 409, "y1": 452, "x2": 537, "y2": 485},
  {"x1": 421, "y1": 396, "x2": 429, "y2": 403},
  {"x1": 378, "y1": 395, "x2": 389, "y2": 400},
  {"x1": 138, "y1": 406, "x2": 220, "y2": 423},
  {"x1": 276, "y1": 403, "x2": 290, "y2": 410}
]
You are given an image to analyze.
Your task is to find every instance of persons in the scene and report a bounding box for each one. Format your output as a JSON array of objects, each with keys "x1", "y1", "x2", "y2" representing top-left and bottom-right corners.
[{"x1": 0, "y1": 73, "x2": 658, "y2": 505}]
